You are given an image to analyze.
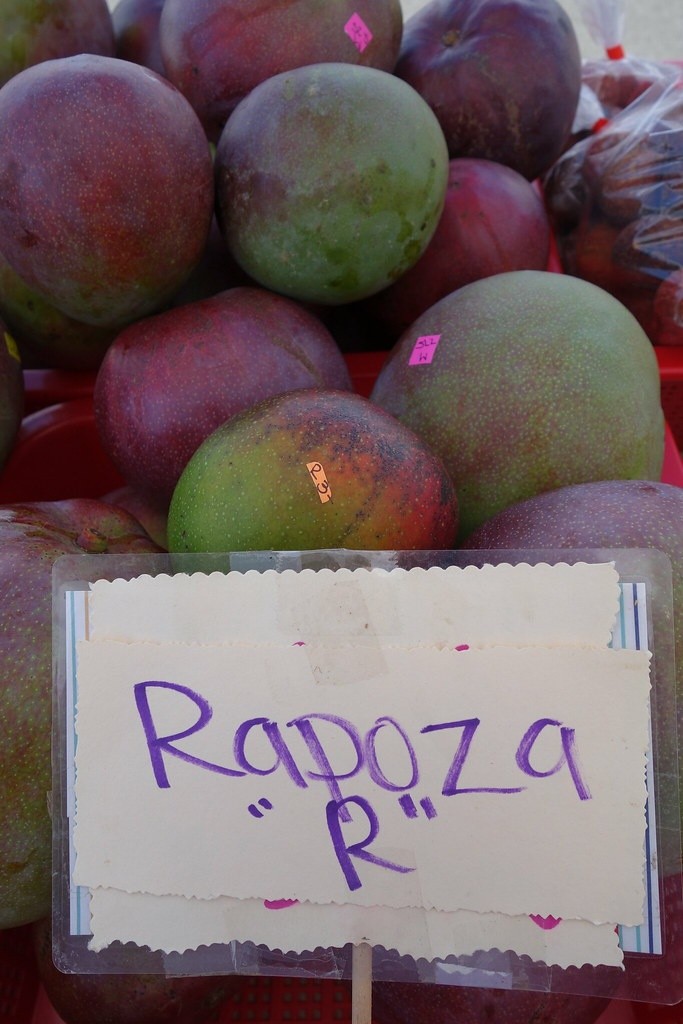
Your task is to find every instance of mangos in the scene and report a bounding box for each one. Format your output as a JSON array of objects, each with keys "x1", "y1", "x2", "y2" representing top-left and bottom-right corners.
[{"x1": 0, "y1": 0, "x2": 683, "y2": 1024}]
